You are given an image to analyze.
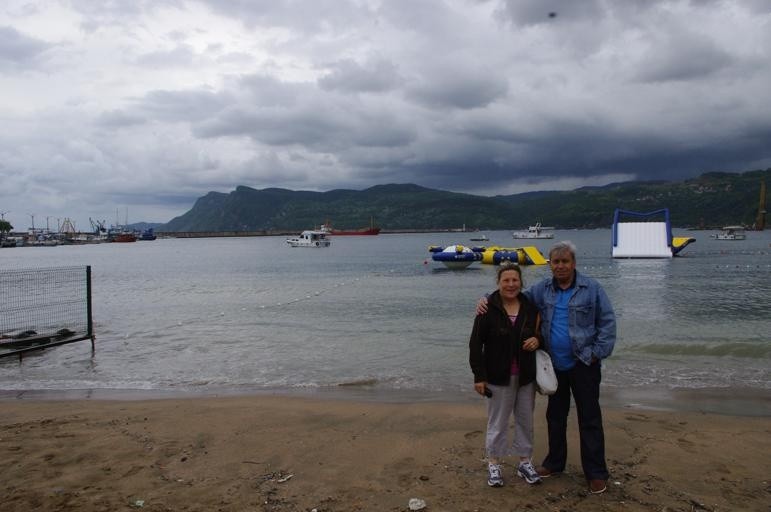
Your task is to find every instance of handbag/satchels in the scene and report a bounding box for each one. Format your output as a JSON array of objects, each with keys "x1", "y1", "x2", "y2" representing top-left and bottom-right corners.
[{"x1": 534, "y1": 313, "x2": 559, "y2": 396}]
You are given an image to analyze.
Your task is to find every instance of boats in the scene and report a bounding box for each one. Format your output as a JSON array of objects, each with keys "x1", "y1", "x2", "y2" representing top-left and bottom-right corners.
[
  {"x1": 469, "y1": 234, "x2": 490, "y2": 242},
  {"x1": 0, "y1": 208, "x2": 157, "y2": 247},
  {"x1": 287, "y1": 220, "x2": 381, "y2": 248},
  {"x1": 428, "y1": 244, "x2": 550, "y2": 269},
  {"x1": 512, "y1": 217, "x2": 555, "y2": 239},
  {"x1": 710, "y1": 225, "x2": 746, "y2": 240}
]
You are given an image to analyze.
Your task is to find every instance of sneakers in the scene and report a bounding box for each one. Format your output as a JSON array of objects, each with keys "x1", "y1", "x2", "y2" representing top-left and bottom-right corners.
[
  {"x1": 517, "y1": 461, "x2": 542, "y2": 484},
  {"x1": 487, "y1": 462, "x2": 504, "y2": 487}
]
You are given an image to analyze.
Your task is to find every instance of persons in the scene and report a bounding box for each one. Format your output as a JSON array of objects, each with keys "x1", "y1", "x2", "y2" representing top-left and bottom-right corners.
[
  {"x1": 469, "y1": 264, "x2": 545, "y2": 488},
  {"x1": 476, "y1": 241, "x2": 616, "y2": 495}
]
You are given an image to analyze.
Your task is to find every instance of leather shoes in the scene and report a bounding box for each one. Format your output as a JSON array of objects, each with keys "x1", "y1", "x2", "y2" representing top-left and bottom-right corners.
[
  {"x1": 588, "y1": 479, "x2": 607, "y2": 494},
  {"x1": 534, "y1": 466, "x2": 564, "y2": 478}
]
